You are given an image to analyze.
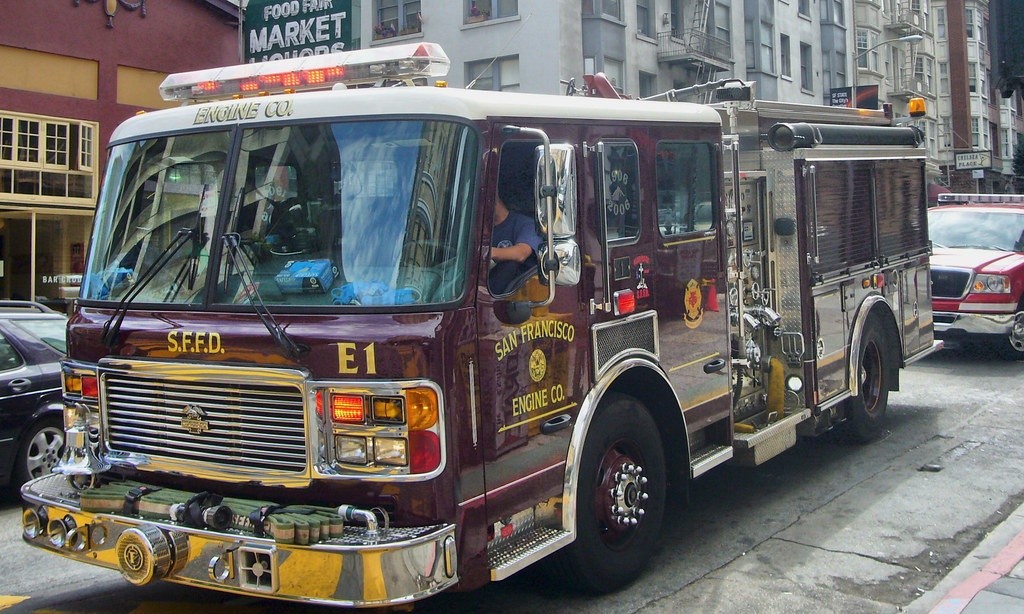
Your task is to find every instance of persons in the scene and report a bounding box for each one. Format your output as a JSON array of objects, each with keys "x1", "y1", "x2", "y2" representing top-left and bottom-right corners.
[{"x1": 439, "y1": 174, "x2": 547, "y2": 297}]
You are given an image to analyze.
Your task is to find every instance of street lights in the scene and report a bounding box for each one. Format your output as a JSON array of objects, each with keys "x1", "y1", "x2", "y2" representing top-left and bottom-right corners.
[{"x1": 852, "y1": 34, "x2": 924, "y2": 110}]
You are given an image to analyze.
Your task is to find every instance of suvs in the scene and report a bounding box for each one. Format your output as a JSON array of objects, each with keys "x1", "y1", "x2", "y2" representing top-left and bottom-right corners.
[
  {"x1": 0, "y1": 300, "x2": 75, "y2": 507},
  {"x1": 924, "y1": 193, "x2": 1024, "y2": 358}
]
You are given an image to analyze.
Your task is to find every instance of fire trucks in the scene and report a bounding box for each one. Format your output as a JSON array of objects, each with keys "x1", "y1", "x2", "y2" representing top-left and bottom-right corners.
[{"x1": 17, "y1": 42, "x2": 948, "y2": 611}]
